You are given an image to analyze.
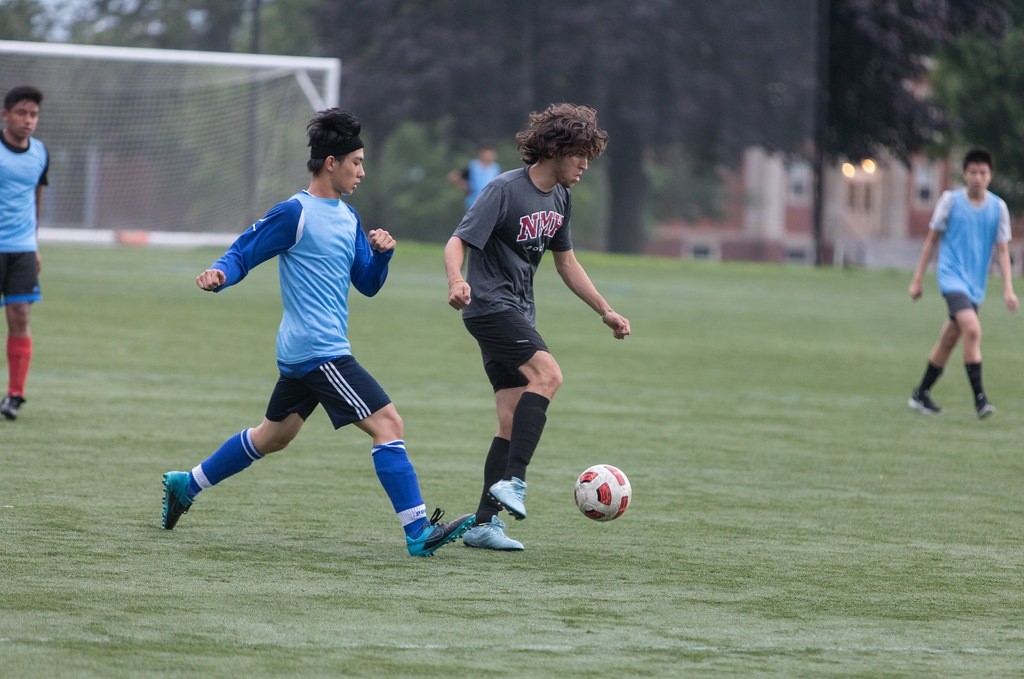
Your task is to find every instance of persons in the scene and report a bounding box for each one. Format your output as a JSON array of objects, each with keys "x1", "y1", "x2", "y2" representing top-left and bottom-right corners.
[
  {"x1": 447, "y1": 139, "x2": 503, "y2": 213},
  {"x1": 908, "y1": 146, "x2": 1018, "y2": 419},
  {"x1": 444, "y1": 101, "x2": 630, "y2": 550},
  {"x1": 163, "y1": 108, "x2": 477, "y2": 556},
  {"x1": 1, "y1": 85, "x2": 50, "y2": 420}
]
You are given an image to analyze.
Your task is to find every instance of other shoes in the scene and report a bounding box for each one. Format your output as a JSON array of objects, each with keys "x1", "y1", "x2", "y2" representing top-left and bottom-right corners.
[
  {"x1": 910, "y1": 394, "x2": 943, "y2": 415},
  {"x1": 977, "y1": 404, "x2": 993, "y2": 419}
]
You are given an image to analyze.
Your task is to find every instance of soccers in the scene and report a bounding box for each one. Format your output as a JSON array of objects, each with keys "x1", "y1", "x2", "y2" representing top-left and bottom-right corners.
[{"x1": 574, "y1": 465, "x2": 632, "y2": 523}]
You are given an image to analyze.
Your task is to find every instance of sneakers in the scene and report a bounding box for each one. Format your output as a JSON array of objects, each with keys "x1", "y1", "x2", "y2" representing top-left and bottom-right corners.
[
  {"x1": 406, "y1": 514, "x2": 476, "y2": 557},
  {"x1": 0, "y1": 395, "x2": 25, "y2": 420},
  {"x1": 162, "y1": 471, "x2": 195, "y2": 530},
  {"x1": 463, "y1": 516, "x2": 526, "y2": 551},
  {"x1": 487, "y1": 480, "x2": 526, "y2": 520}
]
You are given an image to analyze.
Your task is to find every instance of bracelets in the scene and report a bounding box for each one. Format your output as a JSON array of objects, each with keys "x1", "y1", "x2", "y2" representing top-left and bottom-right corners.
[{"x1": 448, "y1": 279, "x2": 464, "y2": 288}]
